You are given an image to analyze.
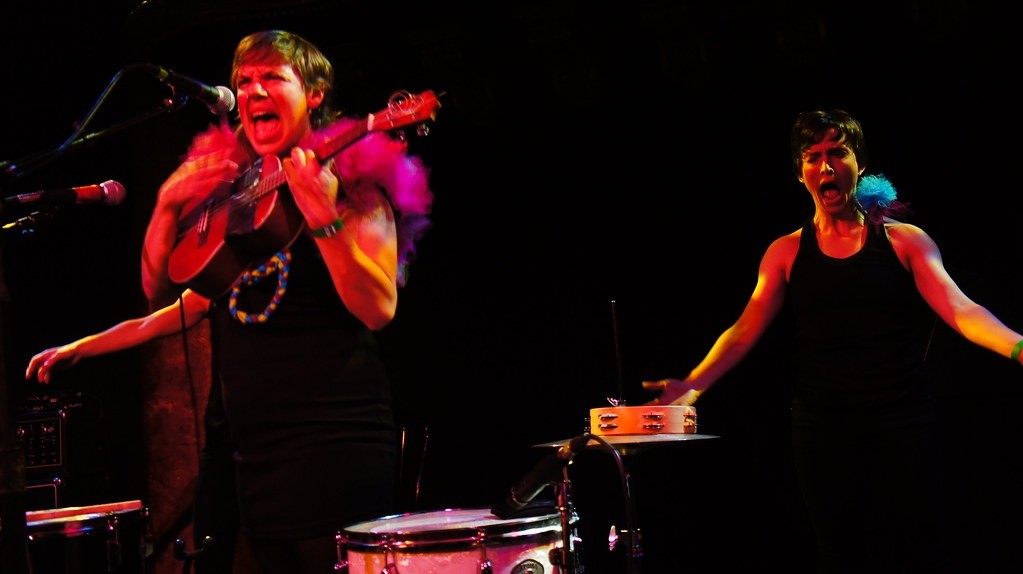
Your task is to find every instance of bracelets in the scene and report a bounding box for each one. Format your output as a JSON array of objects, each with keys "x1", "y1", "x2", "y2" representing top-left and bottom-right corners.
[
  {"x1": 308, "y1": 219, "x2": 344, "y2": 238},
  {"x1": 1011, "y1": 339, "x2": 1023, "y2": 360}
]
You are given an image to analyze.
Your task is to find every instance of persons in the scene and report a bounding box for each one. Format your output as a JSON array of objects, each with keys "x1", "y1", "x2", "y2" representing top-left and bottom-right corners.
[
  {"x1": 641, "y1": 110, "x2": 1023, "y2": 574},
  {"x1": 26, "y1": 28, "x2": 428, "y2": 574}
]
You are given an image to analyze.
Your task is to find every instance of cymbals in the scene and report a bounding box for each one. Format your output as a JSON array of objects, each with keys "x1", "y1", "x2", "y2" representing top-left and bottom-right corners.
[{"x1": 583, "y1": 396, "x2": 701, "y2": 435}]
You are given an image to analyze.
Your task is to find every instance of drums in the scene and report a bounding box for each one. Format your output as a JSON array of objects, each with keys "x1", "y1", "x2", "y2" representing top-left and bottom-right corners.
[
  {"x1": 335, "y1": 506, "x2": 582, "y2": 573},
  {"x1": 26, "y1": 499, "x2": 153, "y2": 574}
]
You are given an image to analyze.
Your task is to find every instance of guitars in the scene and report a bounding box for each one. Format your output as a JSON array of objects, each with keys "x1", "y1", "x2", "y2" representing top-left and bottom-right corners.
[{"x1": 165, "y1": 86, "x2": 442, "y2": 301}]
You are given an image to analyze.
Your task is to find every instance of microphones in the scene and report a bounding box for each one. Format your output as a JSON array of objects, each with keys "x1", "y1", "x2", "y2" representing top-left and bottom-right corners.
[
  {"x1": 5, "y1": 181, "x2": 127, "y2": 207},
  {"x1": 506, "y1": 433, "x2": 591, "y2": 512},
  {"x1": 157, "y1": 68, "x2": 235, "y2": 115}
]
